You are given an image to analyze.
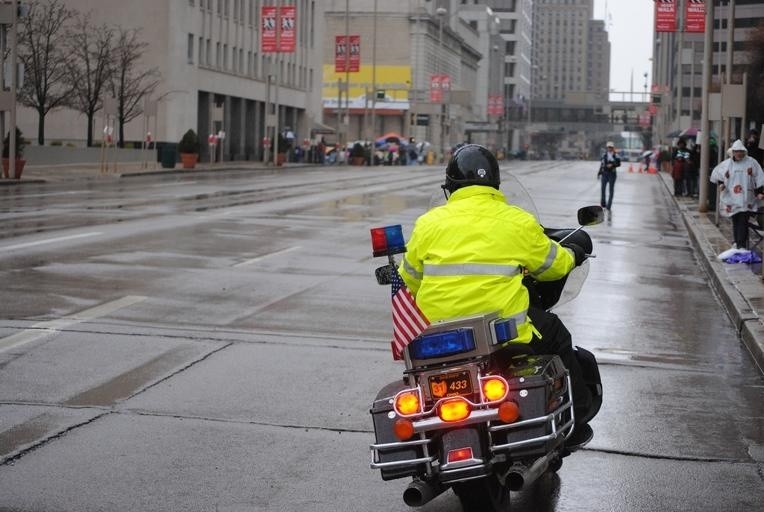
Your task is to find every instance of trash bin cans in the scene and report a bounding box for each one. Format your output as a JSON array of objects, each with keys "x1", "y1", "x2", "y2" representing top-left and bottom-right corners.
[{"x1": 161, "y1": 144, "x2": 176, "y2": 167}]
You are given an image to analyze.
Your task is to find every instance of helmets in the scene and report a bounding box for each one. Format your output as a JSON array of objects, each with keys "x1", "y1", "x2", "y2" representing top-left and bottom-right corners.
[{"x1": 442, "y1": 143, "x2": 502, "y2": 188}]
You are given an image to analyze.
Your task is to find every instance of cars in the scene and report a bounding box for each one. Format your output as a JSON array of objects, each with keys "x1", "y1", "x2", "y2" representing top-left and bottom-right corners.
[{"x1": 635, "y1": 150, "x2": 652, "y2": 162}]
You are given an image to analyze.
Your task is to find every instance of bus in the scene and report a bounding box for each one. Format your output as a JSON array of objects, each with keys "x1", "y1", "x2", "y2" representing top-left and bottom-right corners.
[{"x1": 613, "y1": 149, "x2": 642, "y2": 162}]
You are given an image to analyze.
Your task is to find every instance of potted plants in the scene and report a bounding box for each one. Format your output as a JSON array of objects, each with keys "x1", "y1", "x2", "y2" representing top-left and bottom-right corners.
[
  {"x1": 270, "y1": 133, "x2": 288, "y2": 166},
  {"x1": 177, "y1": 129, "x2": 200, "y2": 168},
  {"x1": 348, "y1": 143, "x2": 366, "y2": 165},
  {"x1": 2, "y1": 126, "x2": 27, "y2": 178}
]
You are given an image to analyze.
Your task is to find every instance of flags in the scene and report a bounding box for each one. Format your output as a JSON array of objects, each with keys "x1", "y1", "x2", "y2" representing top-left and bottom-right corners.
[{"x1": 389, "y1": 258, "x2": 432, "y2": 361}]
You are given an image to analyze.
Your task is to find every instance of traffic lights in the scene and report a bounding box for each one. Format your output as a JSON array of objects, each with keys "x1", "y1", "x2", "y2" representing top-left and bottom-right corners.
[{"x1": 409, "y1": 135, "x2": 415, "y2": 144}]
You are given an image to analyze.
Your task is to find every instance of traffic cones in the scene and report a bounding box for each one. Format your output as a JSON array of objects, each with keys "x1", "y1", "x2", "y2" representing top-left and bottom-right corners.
[
  {"x1": 628, "y1": 163, "x2": 634, "y2": 173},
  {"x1": 637, "y1": 166, "x2": 643, "y2": 173}
]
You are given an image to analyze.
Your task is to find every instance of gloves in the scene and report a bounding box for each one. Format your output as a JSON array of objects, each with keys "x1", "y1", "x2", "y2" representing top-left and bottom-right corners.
[{"x1": 564, "y1": 241, "x2": 586, "y2": 267}]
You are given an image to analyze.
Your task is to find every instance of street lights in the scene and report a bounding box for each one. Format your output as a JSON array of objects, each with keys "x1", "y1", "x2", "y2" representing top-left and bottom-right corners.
[{"x1": 405, "y1": 79, "x2": 417, "y2": 146}]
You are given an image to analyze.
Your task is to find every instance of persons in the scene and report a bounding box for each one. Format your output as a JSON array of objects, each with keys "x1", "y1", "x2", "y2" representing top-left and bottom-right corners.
[
  {"x1": 319, "y1": 138, "x2": 326, "y2": 163},
  {"x1": 645, "y1": 157, "x2": 651, "y2": 172},
  {"x1": 708, "y1": 139, "x2": 764, "y2": 248},
  {"x1": 394, "y1": 143, "x2": 593, "y2": 450},
  {"x1": 654, "y1": 146, "x2": 663, "y2": 171},
  {"x1": 671, "y1": 138, "x2": 698, "y2": 198},
  {"x1": 598, "y1": 141, "x2": 621, "y2": 211}
]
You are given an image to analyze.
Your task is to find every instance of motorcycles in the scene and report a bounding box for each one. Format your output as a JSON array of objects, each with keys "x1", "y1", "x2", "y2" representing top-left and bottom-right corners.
[{"x1": 363, "y1": 170, "x2": 610, "y2": 512}]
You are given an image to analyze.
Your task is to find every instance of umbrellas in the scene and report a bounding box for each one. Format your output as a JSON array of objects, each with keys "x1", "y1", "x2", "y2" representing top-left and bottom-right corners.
[
  {"x1": 640, "y1": 150, "x2": 653, "y2": 159},
  {"x1": 666, "y1": 129, "x2": 682, "y2": 137},
  {"x1": 678, "y1": 127, "x2": 701, "y2": 137}
]
